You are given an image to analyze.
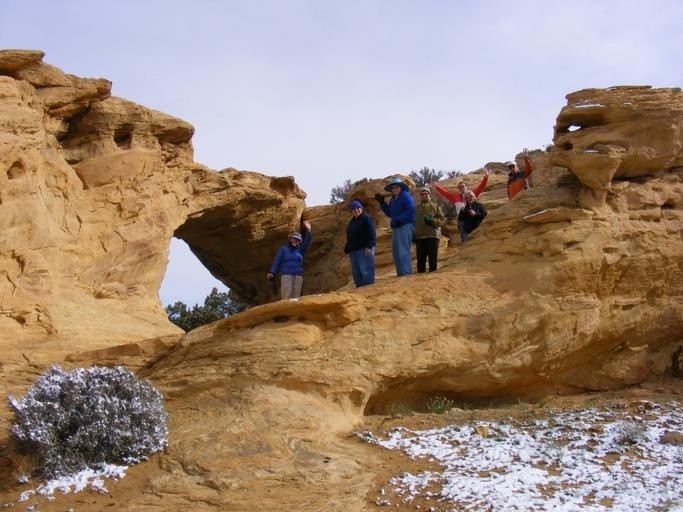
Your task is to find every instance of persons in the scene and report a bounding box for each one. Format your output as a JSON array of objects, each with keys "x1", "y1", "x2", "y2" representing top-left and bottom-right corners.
[
  {"x1": 266, "y1": 219, "x2": 312, "y2": 299},
  {"x1": 410, "y1": 188, "x2": 444, "y2": 273},
  {"x1": 457, "y1": 190, "x2": 488, "y2": 242},
  {"x1": 373, "y1": 177, "x2": 417, "y2": 278},
  {"x1": 432, "y1": 169, "x2": 491, "y2": 243},
  {"x1": 342, "y1": 200, "x2": 376, "y2": 288},
  {"x1": 505, "y1": 147, "x2": 534, "y2": 201}
]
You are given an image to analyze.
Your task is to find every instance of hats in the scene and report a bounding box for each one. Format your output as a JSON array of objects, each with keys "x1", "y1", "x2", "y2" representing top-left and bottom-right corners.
[
  {"x1": 384, "y1": 178, "x2": 409, "y2": 192},
  {"x1": 350, "y1": 200, "x2": 364, "y2": 209},
  {"x1": 506, "y1": 160, "x2": 517, "y2": 167},
  {"x1": 420, "y1": 187, "x2": 430, "y2": 192},
  {"x1": 288, "y1": 232, "x2": 302, "y2": 243}
]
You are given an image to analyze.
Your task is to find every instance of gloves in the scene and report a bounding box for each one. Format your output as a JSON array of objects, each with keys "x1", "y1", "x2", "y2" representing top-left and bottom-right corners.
[
  {"x1": 422, "y1": 214, "x2": 433, "y2": 226},
  {"x1": 375, "y1": 192, "x2": 384, "y2": 203},
  {"x1": 266, "y1": 273, "x2": 274, "y2": 281}
]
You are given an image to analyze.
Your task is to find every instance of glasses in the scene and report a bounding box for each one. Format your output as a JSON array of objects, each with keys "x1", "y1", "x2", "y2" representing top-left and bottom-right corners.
[
  {"x1": 392, "y1": 186, "x2": 398, "y2": 189},
  {"x1": 460, "y1": 185, "x2": 465, "y2": 188},
  {"x1": 352, "y1": 207, "x2": 360, "y2": 211},
  {"x1": 508, "y1": 165, "x2": 515, "y2": 169},
  {"x1": 421, "y1": 193, "x2": 429, "y2": 196}
]
